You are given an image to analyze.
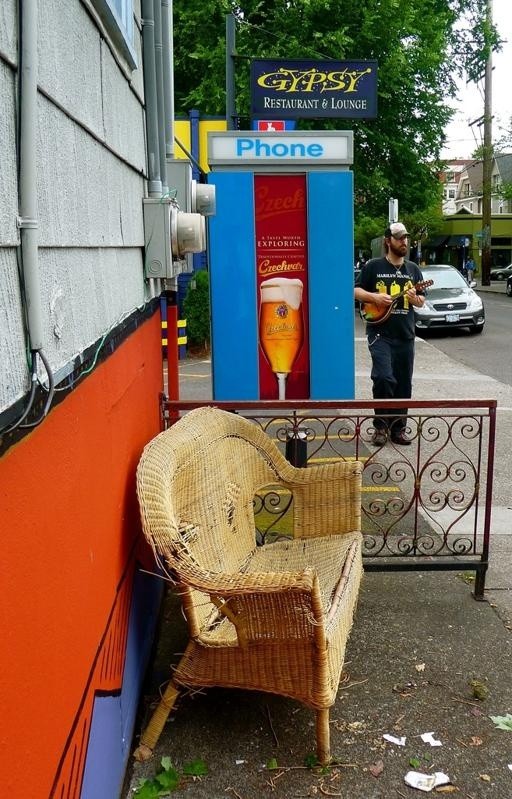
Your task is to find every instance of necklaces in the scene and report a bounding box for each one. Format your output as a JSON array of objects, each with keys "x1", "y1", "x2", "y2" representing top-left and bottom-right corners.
[{"x1": 386, "y1": 256, "x2": 404, "y2": 277}]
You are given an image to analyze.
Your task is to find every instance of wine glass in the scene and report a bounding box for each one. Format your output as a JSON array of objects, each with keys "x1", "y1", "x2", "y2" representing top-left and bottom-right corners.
[{"x1": 259, "y1": 282, "x2": 304, "y2": 404}]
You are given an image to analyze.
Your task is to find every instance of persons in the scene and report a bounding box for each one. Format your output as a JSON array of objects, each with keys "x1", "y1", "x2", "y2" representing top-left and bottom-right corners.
[
  {"x1": 354, "y1": 223, "x2": 428, "y2": 445},
  {"x1": 357, "y1": 252, "x2": 369, "y2": 270},
  {"x1": 466, "y1": 257, "x2": 476, "y2": 282}
]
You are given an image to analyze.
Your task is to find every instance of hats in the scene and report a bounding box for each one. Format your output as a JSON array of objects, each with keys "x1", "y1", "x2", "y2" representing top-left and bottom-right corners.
[{"x1": 384, "y1": 222, "x2": 411, "y2": 239}]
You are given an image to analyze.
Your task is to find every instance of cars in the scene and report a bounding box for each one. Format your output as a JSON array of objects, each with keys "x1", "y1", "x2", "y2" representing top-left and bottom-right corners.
[
  {"x1": 506, "y1": 275, "x2": 512, "y2": 297},
  {"x1": 412, "y1": 265, "x2": 485, "y2": 338},
  {"x1": 490, "y1": 264, "x2": 512, "y2": 281}
]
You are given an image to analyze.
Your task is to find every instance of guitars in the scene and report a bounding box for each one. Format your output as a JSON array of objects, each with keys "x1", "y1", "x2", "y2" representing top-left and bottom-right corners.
[{"x1": 360, "y1": 280, "x2": 434, "y2": 324}]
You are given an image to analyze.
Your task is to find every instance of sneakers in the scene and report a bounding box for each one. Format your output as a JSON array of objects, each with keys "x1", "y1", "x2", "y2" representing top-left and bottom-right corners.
[
  {"x1": 372, "y1": 428, "x2": 389, "y2": 446},
  {"x1": 391, "y1": 430, "x2": 412, "y2": 445}
]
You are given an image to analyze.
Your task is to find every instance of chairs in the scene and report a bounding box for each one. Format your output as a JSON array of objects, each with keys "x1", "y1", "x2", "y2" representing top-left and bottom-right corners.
[{"x1": 132, "y1": 406, "x2": 366, "y2": 768}]
